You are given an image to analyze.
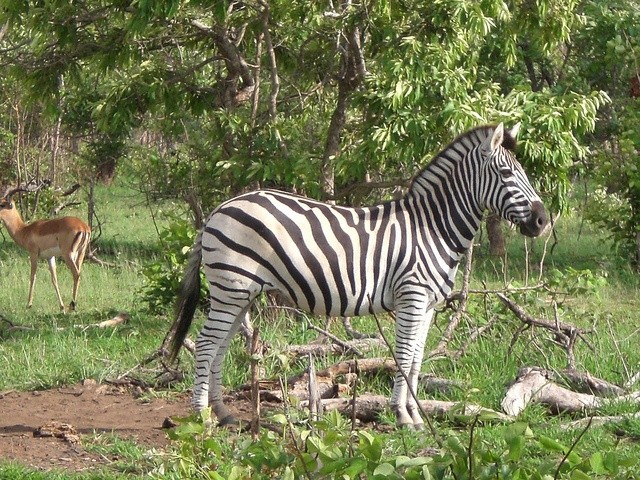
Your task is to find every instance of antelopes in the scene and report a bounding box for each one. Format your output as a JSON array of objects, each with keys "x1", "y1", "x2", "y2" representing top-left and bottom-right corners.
[{"x1": 0, "y1": 177, "x2": 92, "y2": 314}]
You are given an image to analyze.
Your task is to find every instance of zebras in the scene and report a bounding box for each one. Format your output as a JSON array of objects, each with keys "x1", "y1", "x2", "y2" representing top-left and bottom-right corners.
[{"x1": 157, "y1": 120, "x2": 549, "y2": 436}]
